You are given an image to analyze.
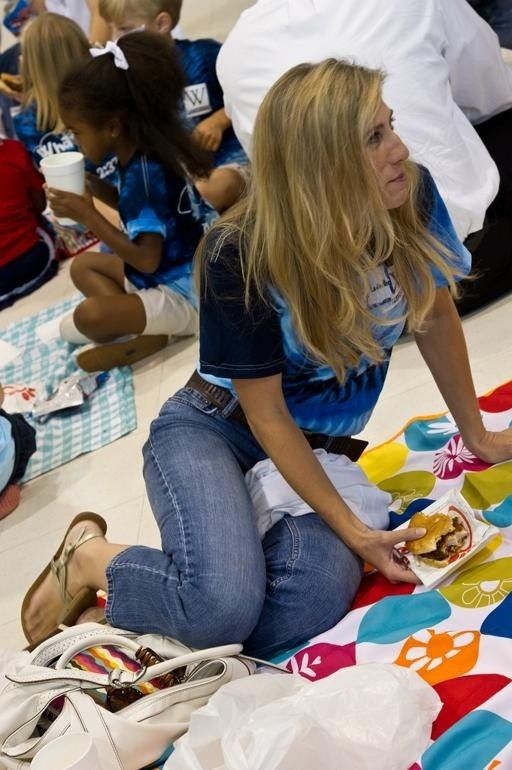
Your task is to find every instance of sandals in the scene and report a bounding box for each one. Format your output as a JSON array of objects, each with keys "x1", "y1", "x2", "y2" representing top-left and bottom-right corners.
[{"x1": 18, "y1": 513, "x2": 111, "y2": 651}]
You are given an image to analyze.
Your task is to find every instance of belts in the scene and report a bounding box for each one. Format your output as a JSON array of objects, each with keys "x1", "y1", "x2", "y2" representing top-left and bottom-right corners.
[{"x1": 188, "y1": 370, "x2": 367, "y2": 464}]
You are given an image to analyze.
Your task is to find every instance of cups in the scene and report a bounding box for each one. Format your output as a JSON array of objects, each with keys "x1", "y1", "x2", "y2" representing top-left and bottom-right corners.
[{"x1": 41, "y1": 152, "x2": 84, "y2": 227}]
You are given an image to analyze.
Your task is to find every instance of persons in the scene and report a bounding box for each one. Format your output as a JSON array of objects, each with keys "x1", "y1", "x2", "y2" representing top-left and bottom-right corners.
[
  {"x1": 216, "y1": 0, "x2": 510, "y2": 322},
  {"x1": 19, "y1": 56, "x2": 512, "y2": 657},
  {"x1": 1, "y1": 0, "x2": 239, "y2": 371},
  {"x1": 3, "y1": 390, "x2": 38, "y2": 521}
]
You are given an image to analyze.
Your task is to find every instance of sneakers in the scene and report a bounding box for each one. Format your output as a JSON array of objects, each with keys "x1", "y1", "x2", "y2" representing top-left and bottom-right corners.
[
  {"x1": 59, "y1": 312, "x2": 169, "y2": 373},
  {"x1": 0, "y1": 486, "x2": 21, "y2": 519}
]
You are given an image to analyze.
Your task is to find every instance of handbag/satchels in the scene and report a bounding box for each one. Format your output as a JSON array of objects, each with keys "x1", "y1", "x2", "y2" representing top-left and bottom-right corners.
[{"x1": 1, "y1": 622, "x2": 259, "y2": 769}]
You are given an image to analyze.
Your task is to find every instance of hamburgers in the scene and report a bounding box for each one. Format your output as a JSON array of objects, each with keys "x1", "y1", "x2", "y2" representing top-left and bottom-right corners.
[{"x1": 409, "y1": 512, "x2": 473, "y2": 566}]
